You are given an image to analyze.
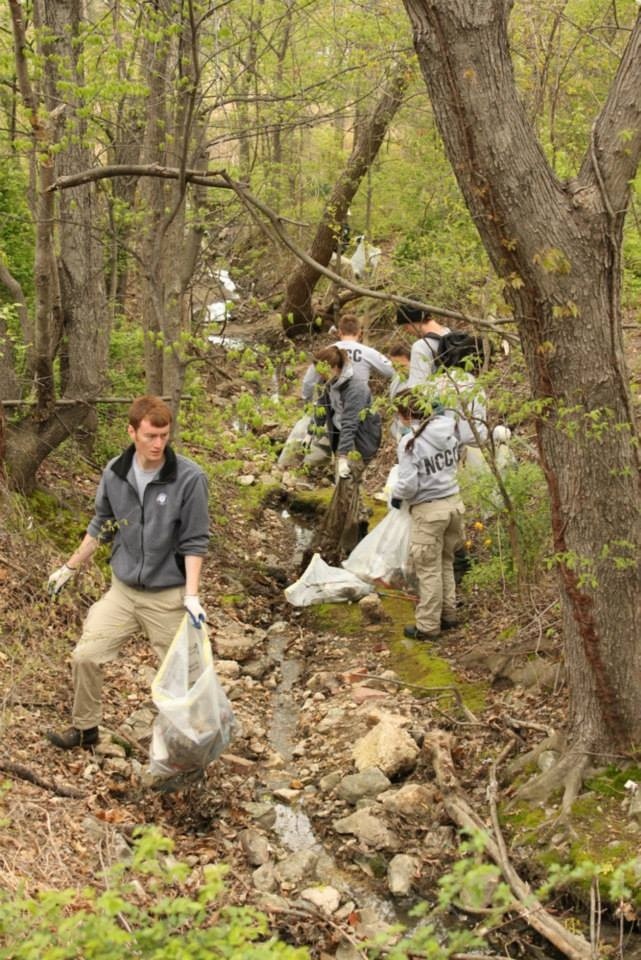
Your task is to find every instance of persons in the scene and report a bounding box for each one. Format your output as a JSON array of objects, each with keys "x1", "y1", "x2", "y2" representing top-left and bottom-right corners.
[
  {"x1": 47, "y1": 396, "x2": 211, "y2": 749},
  {"x1": 397, "y1": 303, "x2": 489, "y2": 401},
  {"x1": 301, "y1": 314, "x2": 395, "y2": 399},
  {"x1": 312, "y1": 346, "x2": 382, "y2": 517},
  {"x1": 391, "y1": 387, "x2": 512, "y2": 641}
]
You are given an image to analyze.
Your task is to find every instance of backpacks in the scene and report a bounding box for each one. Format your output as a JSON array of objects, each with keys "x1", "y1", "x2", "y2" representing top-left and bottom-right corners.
[{"x1": 424, "y1": 327, "x2": 494, "y2": 376}]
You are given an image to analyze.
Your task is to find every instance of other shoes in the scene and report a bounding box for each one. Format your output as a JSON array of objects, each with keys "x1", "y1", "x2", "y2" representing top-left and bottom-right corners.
[
  {"x1": 441, "y1": 619, "x2": 456, "y2": 630},
  {"x1": 404, "y1": 626, "x2": 436, "y2": 640}
]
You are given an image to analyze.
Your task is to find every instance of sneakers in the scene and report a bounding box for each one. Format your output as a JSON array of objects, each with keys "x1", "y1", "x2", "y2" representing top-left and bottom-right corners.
[{"x1": 48, "y1": 727, "x2": 99, "y2": 747}]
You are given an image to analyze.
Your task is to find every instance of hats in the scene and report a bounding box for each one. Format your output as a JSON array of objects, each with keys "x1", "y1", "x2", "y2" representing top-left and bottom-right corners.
[{"x1": 397, "y1": 302, "x2": 428, "y2": 324}]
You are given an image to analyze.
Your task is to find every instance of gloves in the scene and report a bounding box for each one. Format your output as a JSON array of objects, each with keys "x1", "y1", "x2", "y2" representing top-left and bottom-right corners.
[
  {"x1": 391, "y1": 499, "x2": 402, "y2": 508},
  {"x1": 46, "y1": 563, "x2": 77, "y2": 595},
  {"x1": 302, "y1": 435, "x2": 312, "y2": 449},
  {"x1": 183, "y1": 596, "x2": 207, "y2": 629},
  {"x1": 338, "y1": 455, "x2": 352, "y2": 478}
]
